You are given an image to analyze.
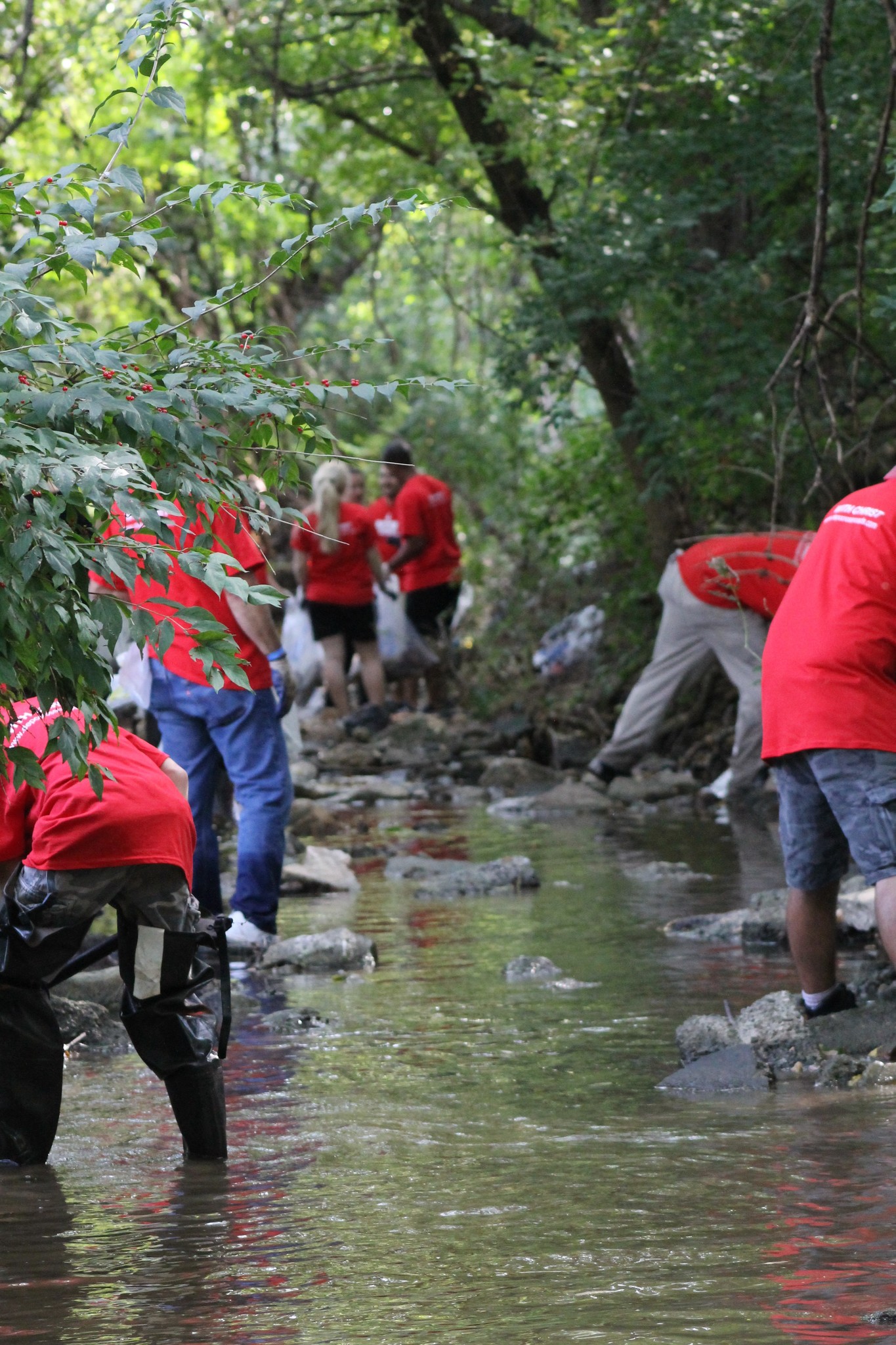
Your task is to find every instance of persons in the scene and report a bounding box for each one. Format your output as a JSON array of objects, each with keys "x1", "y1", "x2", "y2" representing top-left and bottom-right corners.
[
  {"x1": 759, "y1": 464, "x2": 894, "y2": 1017},
  {"x1": 87, "y1": 401, "x2": 300, "y2": 960},
  {"x1": 291, "y1": 436, "x2": 462, "y2": 715},
  {"x1": 1, "y1": 693, "x2": 230, "y2": 1163},
  {"x1": 580, "y1": 530, "x2": 820, "y2": 798}
]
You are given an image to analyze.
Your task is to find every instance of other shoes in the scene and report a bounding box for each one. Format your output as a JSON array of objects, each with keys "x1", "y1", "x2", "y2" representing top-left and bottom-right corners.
[
  {"x1": 225, "y1": 909, "x2": 281, "y2": 949},
  {"x1": 798, "y1": 984, "x2": 859, "y2": 1019},
  {"x1": 582, "y1": 757, "x2": 621, "y2": 795}
]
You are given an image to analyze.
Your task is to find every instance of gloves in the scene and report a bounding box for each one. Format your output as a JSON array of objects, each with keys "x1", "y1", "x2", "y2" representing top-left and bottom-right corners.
[{"x1": 267, "y1": 646, "x2": 297, "y2": 724}]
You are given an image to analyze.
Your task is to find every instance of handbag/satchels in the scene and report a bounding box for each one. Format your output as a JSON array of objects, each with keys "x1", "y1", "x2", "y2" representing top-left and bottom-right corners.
[
  {"x1": 278, "y1": 585, "x2": 322, "y2": 708},
  {"x1": 371, "y1": 573, "x2": 408, "y2": 663}
]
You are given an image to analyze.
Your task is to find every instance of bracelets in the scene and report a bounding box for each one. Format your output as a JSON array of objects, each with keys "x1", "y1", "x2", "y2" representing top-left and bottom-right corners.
[{"x1": 268, "y1": 649, "x2": 285, "y2": 661}]
